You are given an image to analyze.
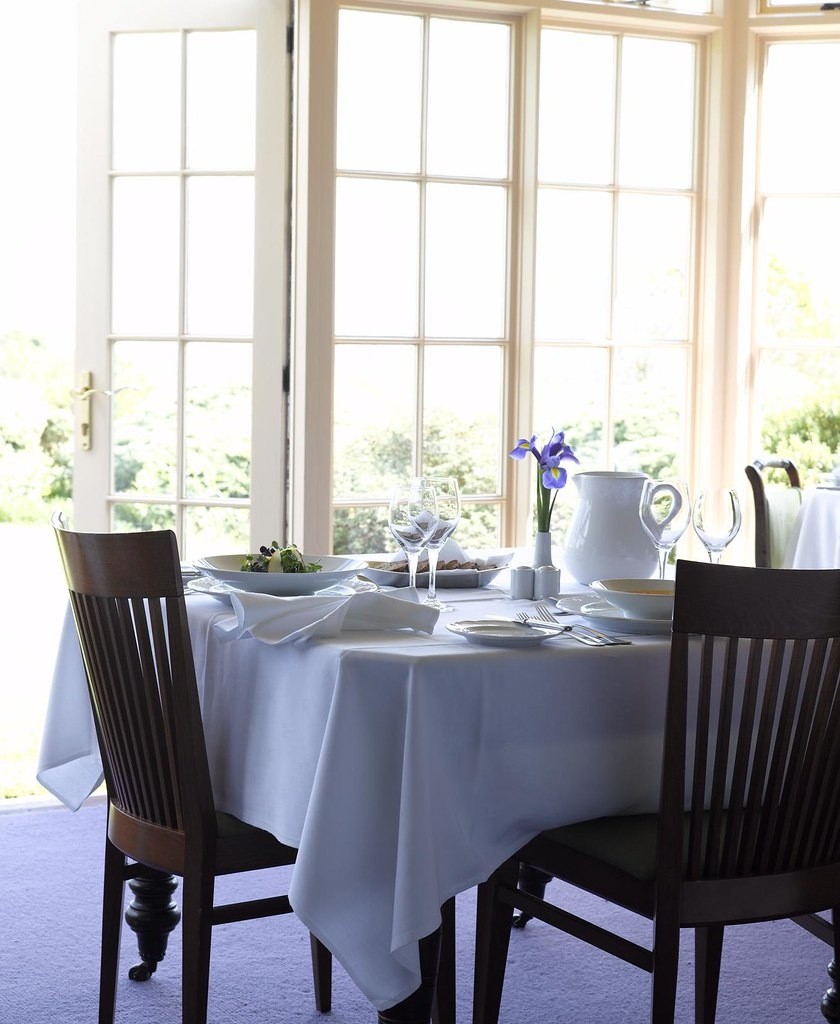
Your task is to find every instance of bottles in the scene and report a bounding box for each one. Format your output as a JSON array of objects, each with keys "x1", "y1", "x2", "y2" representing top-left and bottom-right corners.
[
  {"x1": 511, "y1": 565, "x2": 535, "y2": 600},
  {"x1": 535, "y1": 565, "x2": 560, "y2": 600}
]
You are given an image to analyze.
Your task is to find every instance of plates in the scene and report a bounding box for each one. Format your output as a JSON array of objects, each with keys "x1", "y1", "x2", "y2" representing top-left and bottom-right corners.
[
  {"x1": 445, "y1": 620, "x2": 563, "y2": 647},
  {"x1": 187, "y1": 576, "x2": 378, "y2": 608},
  {"x1": 589, "y1": 579, "x2": 675, "y2": 618},
  {"x1": 556, "y1": 595, "x2": 674, "y2": 634},
  {"x1": 192, "y1": 554, "x2": 369, "y2": 595},
  {"x1": 362, "y1": 560, "x2": 514, "y2": 589}
]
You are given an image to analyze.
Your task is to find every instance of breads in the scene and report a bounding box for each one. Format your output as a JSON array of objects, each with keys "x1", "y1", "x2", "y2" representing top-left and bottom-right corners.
[{"x1": 376, "y1": 560, "x2": 478, "y2": 572}]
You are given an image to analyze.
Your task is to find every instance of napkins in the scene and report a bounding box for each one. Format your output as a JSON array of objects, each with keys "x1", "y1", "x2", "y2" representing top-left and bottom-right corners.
[{"x1": 212, "y1": 586, "x2": 439, "y2": 648}]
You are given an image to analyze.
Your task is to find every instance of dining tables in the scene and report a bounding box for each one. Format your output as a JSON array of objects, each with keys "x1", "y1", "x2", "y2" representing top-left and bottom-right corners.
[{"x1": 36, "y1": 546, "x2": 840, "y2": 1024}]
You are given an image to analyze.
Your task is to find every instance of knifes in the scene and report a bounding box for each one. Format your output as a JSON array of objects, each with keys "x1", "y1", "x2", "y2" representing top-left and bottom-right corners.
[{"x1": 484, "y1": 614, "x2": 572, "y2": 631}]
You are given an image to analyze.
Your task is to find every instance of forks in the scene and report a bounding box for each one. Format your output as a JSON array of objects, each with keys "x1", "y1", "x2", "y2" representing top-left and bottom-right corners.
[{"x1": 516, "y1": 605, "x2": 623, "y2": 645}]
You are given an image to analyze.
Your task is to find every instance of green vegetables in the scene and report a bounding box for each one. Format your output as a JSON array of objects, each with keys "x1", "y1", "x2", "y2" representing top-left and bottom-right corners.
[{"x1": 241, "y1": 541, "x2": 322, "y2": 573}]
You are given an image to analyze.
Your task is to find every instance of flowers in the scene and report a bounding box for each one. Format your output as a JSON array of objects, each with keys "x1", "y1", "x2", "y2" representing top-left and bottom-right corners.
[{"x1": 509, "y1": 426, "x2": 580, "y2": 533}]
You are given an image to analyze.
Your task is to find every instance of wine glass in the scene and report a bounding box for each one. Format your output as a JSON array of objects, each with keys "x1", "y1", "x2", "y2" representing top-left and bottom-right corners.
[
  {"x1": 692, "y1": 490, "x2": 741, "y2": 564},
  {"x1": 420, "y1": 479, "x2": 461, "y2": 613},
  {"x1": 389, "y1": 477, "x2": 439, "y2": 587},
  {"x1": 639, "y1": 479, "x2": 691, "y2": 579}
]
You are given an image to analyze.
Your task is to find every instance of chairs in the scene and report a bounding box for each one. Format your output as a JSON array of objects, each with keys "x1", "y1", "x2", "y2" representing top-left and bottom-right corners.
[
  {"x1": 745, "y1": 459, "x2": 805, "y2": 570},
  {"x1": 49, "y1": 509, "x2": 457, "y2": 1024},
  {"x1": 473, "y1": 559, "x2": 840, "y2": 1024}
]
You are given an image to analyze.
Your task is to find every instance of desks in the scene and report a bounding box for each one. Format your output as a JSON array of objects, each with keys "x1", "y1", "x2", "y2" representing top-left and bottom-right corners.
[{"x1": 783, "y1": 486, "x2": 840, "y2": 569}]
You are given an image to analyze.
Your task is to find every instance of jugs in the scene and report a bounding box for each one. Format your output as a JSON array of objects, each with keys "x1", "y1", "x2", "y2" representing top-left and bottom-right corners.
[{"x1": 567, "y1": 471, "x2": 682, "y2": 585}]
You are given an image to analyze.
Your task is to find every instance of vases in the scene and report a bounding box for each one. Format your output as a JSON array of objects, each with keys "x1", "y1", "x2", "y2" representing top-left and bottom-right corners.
[{"x1": 531, "y1": 531, "x2": 561, "y2": 594}]
